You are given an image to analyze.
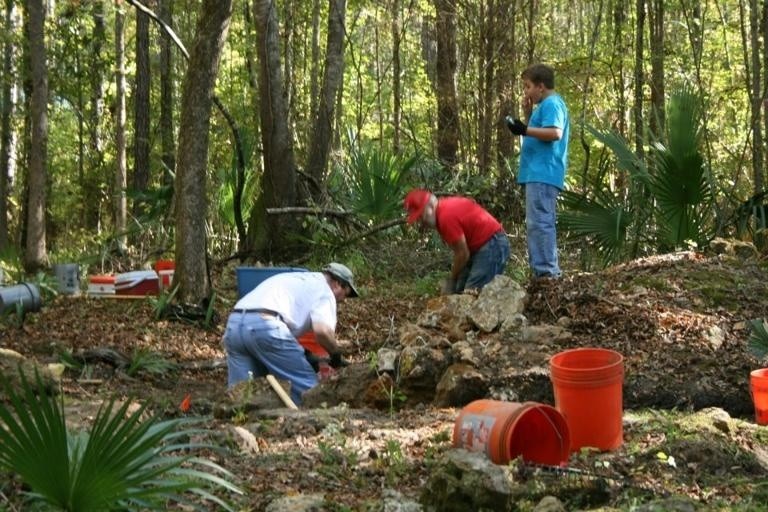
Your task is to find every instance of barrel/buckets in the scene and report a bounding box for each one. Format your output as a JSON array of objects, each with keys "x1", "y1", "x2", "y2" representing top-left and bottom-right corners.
[
  {"x1": 57, "y1": 263, "x2": 79, "y2": 295},
  {"x1": 298, "y1": 323, "x2": 329, "y2": 359},
  {"x1": 750, "y1": 370, "x2": 768, "y2": 423},
  {"x1": 0, "y1": 282, "x2": 40, "y2": 314},
  {"x1": 236, "y1": 266, "x2": 310, "y2": 294},
  {"x1": 156, "y1": 260, "x2": 174, "y2": 287},
  {"x1": 549, "y1": 348, "x2": 624, "y2": 453},
  {"x1": 454, "y1": 399, "x2": 569, "y2": 469}
]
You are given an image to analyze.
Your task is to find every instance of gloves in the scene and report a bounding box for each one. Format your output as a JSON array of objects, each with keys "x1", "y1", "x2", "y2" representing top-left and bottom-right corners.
[{"x1": 508, "y1": 119, "x2": 527, "y2": 136}]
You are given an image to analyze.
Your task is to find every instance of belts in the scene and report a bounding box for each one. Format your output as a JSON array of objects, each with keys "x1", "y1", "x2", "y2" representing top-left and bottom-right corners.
[{"x1": 233, "y1": 310, "x2": 283, "y2": 319}]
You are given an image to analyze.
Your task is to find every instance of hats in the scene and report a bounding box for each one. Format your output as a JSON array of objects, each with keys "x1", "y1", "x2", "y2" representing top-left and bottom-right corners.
[
  {"x1": 322, "y1": 262, "x2": 362, "y2": 297},
  {"x1": 404, "y1": 190, "x2": 431, "y2": 224}
]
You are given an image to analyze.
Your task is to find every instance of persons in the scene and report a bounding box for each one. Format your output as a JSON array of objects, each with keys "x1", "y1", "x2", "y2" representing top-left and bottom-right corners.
[
  {"x1": 504, "y1": 63, "x2": 570, "y2": 280},
  {"x1": 224, "y1": 260, "x2": 362, "y2": 408},
  {"x1": 403, "y1": 188, "x2": 513, "y2": 296}
]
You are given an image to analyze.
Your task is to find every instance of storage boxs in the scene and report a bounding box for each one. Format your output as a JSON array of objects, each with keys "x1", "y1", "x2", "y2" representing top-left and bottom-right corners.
[
  {"x1": 115, "y1": 270, "x2": 159, "y2": 295},
  {"x1": 237, "y1": 267, "x2": 309, "y2": 300}
]
